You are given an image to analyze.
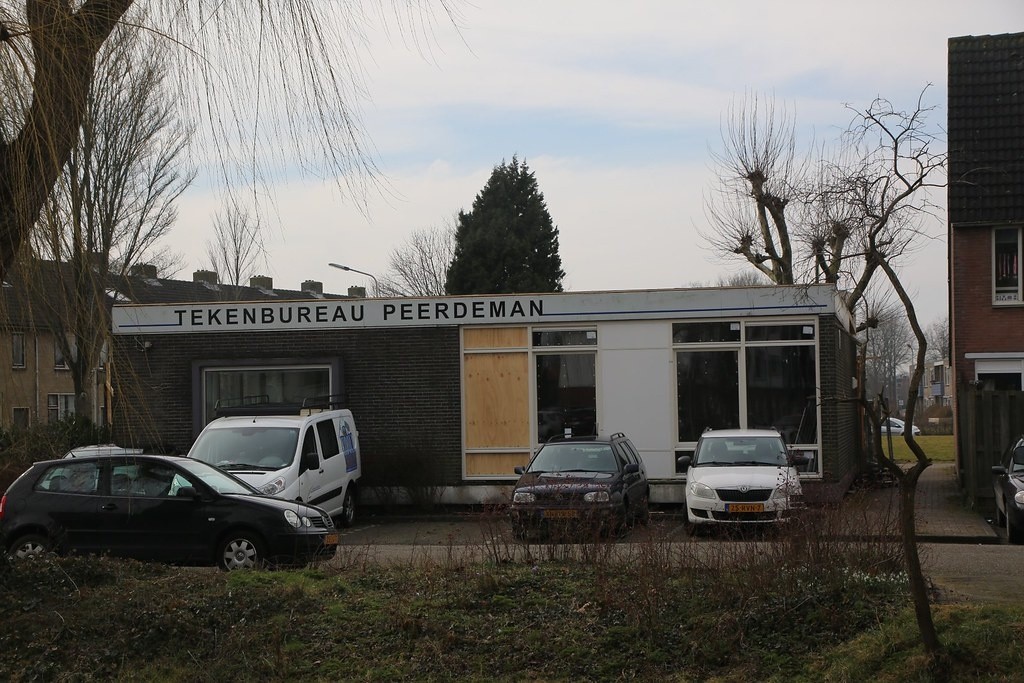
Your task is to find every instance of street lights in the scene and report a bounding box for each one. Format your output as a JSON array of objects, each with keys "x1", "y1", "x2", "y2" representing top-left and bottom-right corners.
[{"x1": 907, "y1": 344, "x2": 914, "y2": 377}]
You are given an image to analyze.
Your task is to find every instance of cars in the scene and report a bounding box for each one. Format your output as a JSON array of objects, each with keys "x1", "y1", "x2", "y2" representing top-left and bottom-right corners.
[
  {"x1": 511, "y1": 434, "x2": 650, "y2": 539},
  {"x1": 678, "y1": 427, "x2": 809, "y2": 540},
  {"x1": 992, "y1": 435, "x2": 1024, "y2": 545},
  {"x1": 870, "y1": 417, "x2": 921, "y2": 437}
]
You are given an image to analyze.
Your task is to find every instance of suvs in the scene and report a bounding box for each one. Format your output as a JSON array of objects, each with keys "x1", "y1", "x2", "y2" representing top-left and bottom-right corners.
[
  {"x1": 169, "y1": 394, "x2": 363, "y2": 528},
  {"x1": 0, "y1": 445, "x2": 339, "y2": 573}
]
